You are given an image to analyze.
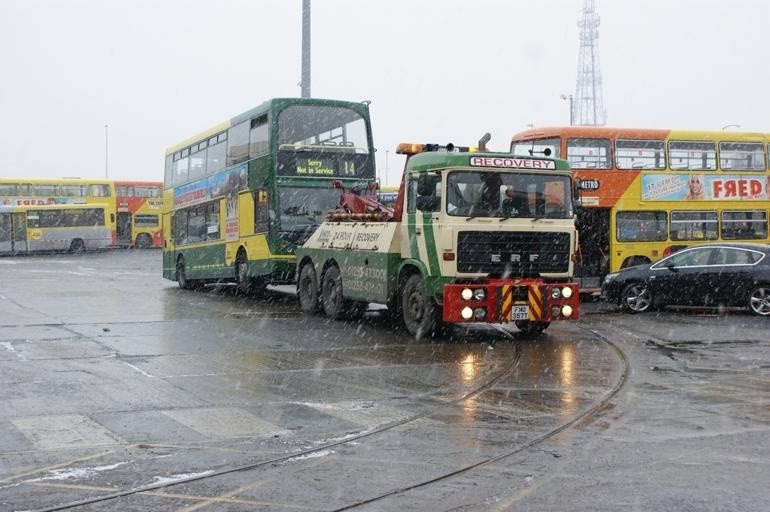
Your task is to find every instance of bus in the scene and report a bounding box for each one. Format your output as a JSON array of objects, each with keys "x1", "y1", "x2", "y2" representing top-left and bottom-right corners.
[
  {"x1": 0, "y1": 176, "x2": 163, "y2": 254},
  {"x1": 377, "y1": 183, "x2": 401, "y2": 210},
  {"x1": 159, "y1": 96, "x2": 380, "y2": 294},
  {"x1": 509, "y1": 126, "x2": 769, "y2": 293}
]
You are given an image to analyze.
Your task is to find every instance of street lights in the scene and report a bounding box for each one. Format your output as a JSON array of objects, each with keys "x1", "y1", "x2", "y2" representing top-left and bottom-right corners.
[
  {"x1": 560, "y1": 92, "x2": 574, "y2": 125},
  {"x1": 721, "y1": 123, "x2": 741, "y2": 130},
  {"x1": 105, "y1": 123, "x2": 110, "y2": 179},
  {"x1": 384, "y1": 148, "x2": 390, "y2": 186}
]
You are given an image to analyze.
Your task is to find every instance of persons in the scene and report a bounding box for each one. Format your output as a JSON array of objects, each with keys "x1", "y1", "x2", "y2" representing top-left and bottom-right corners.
[{"x1": 681, "y1": 175, "x2": 707, "y2": 201}]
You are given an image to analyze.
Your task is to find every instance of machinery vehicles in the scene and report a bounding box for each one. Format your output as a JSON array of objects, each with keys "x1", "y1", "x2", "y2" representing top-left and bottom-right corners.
[{"x1": 292, "y1": 130, "x2": 582, "y2": 339}]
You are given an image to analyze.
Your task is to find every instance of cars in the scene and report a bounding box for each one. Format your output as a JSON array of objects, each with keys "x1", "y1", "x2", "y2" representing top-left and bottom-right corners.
[{"x1": 598, "y1": 243, "x2": 770, "y2": 316}]
